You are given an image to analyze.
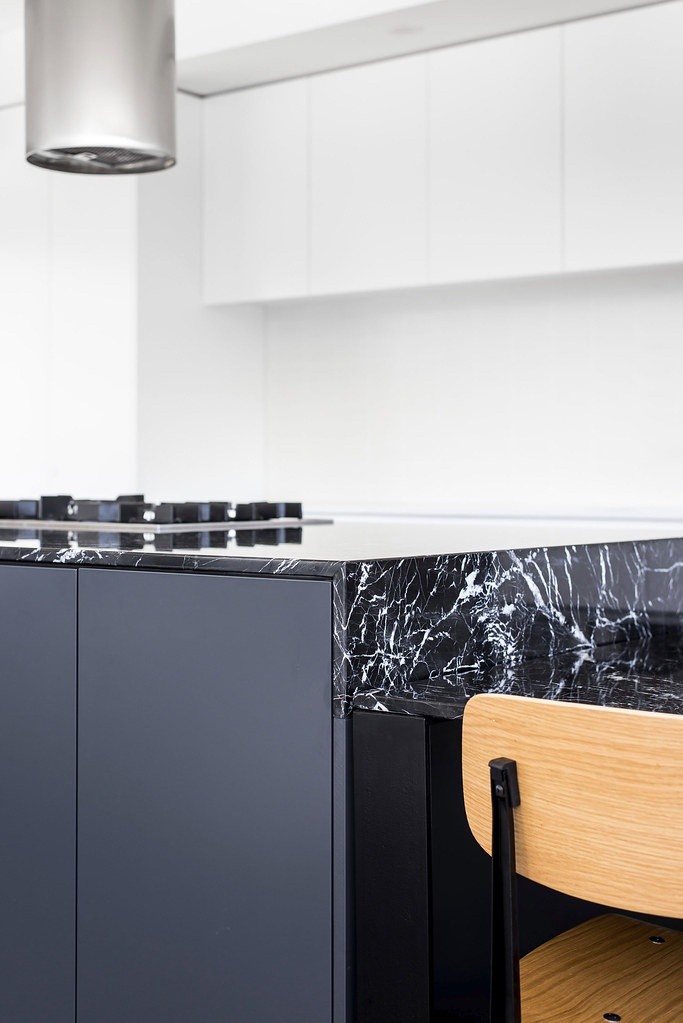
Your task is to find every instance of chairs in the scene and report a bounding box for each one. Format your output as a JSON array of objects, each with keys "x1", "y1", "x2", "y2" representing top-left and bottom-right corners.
[{"x1": 458, "y1": 692, "x2": 683, "y2": 1023}]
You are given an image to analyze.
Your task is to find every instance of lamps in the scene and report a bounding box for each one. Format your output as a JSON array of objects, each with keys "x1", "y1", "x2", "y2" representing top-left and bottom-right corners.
[{"x1": 24, "y1": 0, "x2": 177, "y2": 175}]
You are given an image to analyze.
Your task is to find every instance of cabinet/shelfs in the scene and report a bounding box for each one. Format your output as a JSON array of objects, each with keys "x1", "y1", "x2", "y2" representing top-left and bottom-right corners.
[
  {"x1": 0, "y1": 560, "x2": 508, "y2": 1023},
  {"x1": 193, "y1": 0, "x2": 683, "y2": 309}
]
